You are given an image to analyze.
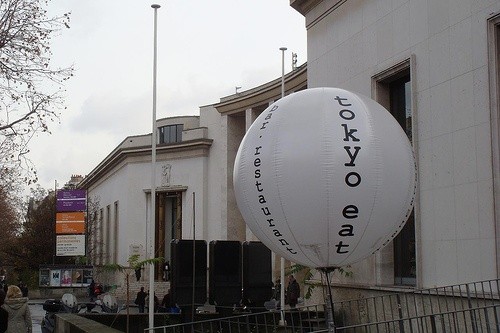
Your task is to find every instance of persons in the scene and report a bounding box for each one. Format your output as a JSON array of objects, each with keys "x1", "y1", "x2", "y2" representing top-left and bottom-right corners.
[
  {"x1": 272, "y1": 274, "x2": 301, "y2": 308},
  {"x1": 88, "y1": 280, "x2": 105, "y2": 302},
  {"x1": 137, "y1": 287, "x2": 158, "y2": 313},
  {"x1": 0, "y1": 281, "x2": 32, "y2": 333}
]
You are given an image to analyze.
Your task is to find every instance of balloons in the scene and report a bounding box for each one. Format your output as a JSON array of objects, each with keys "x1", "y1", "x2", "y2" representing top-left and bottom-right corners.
[{"x1": 231, "y1": 87, "x2": 417, "y2": 272}]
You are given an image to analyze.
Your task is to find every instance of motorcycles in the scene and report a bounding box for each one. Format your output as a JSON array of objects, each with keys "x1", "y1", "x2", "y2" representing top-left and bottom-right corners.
[
  {"x1": 94, "y1": 288, "x2": 102, "y2": 295},
  {"x1": 41, "y1": 294, "x2": 86, "y2": 333},
  {"x1": 85, "y1": 295, "x2": 126, "y2": 314}
]
